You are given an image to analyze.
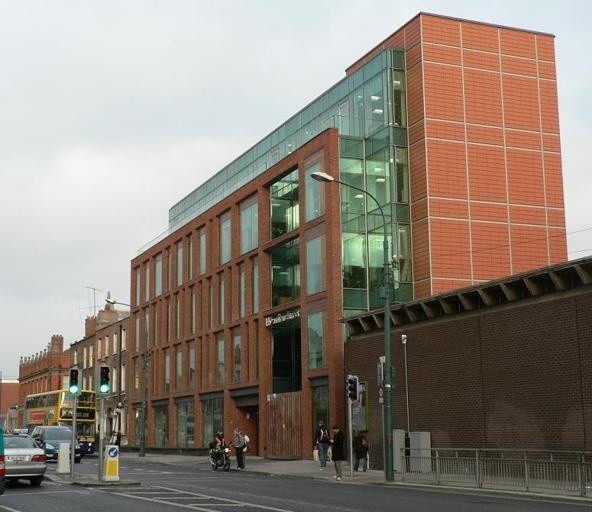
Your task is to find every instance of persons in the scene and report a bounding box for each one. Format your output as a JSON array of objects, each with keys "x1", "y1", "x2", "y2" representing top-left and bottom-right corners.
[
  {"x1": 228, "y1": 426, "x2": 248, "y2": 469},
  {"x1": 328, "y1": 424, "x2": 345, "y2": 481},
  {"x1": 316, "y1": 420, "x2": 331, "y2": 468},
  {"x1": 214, "y1": 430, "x2": 231, "y2": 464},
  {"x1": 352, "y1": 426, "x2": 369, "y2": 472}
]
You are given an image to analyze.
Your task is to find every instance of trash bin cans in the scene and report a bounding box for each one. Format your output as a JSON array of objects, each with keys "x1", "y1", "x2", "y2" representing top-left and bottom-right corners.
[
  {"x1": 102, "y1": 444, "x2": 119, "y2": 481},
  {"x1": 57, "y1": 442, "x2": 71, "y2": 474}
]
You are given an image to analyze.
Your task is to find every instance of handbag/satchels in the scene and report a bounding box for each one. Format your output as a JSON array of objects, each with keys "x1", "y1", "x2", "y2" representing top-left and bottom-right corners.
[{"x1": 314, "y1": 450, "x2": 319, "y2": 460}]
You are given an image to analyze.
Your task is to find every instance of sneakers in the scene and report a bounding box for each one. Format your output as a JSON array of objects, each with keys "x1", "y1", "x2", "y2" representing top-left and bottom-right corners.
[{"x1": 337, "y1": 476, "x2": 341, "y2": 481}]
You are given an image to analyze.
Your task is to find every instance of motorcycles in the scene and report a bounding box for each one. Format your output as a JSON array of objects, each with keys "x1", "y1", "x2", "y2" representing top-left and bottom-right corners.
[{"x1": 208, "y1": 441, "x2": 234, "y2": 471}]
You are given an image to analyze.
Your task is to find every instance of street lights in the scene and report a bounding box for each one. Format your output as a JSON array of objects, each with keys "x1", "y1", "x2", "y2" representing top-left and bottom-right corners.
[
  {"x1": 310, "y1": 171, "x2": 395, "y2": 481},
  {"x1": 105, "y1": 298, "x2": 151, "y2": 457}
]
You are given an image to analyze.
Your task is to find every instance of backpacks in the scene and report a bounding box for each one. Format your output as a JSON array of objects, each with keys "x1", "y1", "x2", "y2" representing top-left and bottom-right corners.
[{"x1": 320, "y1": 429, "x2": 329, "y2": 441}]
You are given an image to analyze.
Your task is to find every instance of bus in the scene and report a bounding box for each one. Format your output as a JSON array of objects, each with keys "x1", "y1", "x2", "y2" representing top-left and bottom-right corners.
[{"x1": 24, "y1": 390, "x2": 97, "y2": 462}]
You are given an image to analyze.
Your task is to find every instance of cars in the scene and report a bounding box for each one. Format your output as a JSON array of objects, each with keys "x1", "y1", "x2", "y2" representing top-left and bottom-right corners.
[
  {"x1": 32, "y1": 425, "x2": 82, "y2": 463},
  {"x1": 3, "y1": 434, "x2": 48, "y2": 487}
]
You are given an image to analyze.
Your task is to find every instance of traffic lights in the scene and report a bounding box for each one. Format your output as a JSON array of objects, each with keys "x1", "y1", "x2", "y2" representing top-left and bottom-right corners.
[
  {"x1": 99, "y1": 364, "x2": 111, "y2": 395},
  {"x1": 69, "y1": 367, "x2": 83, "y2": 396},
  {"x1": 346, "y1": 375, "x2": 360, "y2": 404}
]
